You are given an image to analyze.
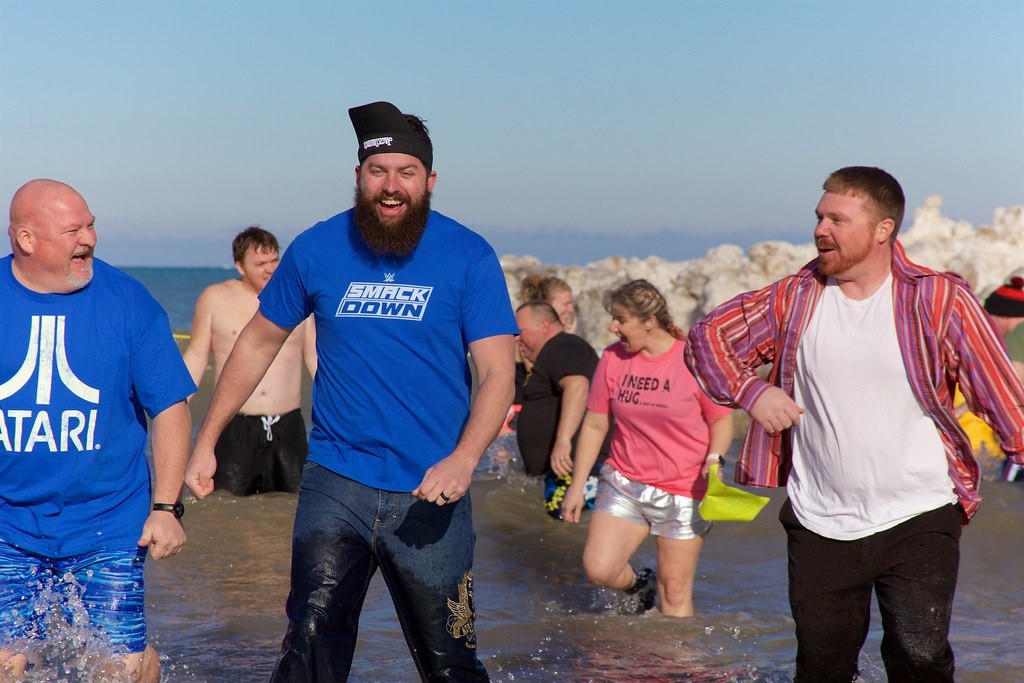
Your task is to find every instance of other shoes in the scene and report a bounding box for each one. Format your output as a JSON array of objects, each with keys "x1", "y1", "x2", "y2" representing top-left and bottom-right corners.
[{"x1": 626, "y1": 567, "x2": 657, "y2": 611}]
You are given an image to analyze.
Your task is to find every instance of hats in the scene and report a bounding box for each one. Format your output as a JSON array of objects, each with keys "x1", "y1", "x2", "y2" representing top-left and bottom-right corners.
[
  {"x1": 983, "y1": 276, "x2": 1024, "y2": 317},
  {"x1": 348, "y1": 101, "x2": 433, "y2": 172}
]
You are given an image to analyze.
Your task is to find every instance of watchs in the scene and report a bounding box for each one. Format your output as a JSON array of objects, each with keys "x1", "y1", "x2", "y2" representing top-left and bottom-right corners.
[
  {"x1": 153, "y1": 501, "x2": 184, "y2": 519},
  {"x1": 706, "y1": 453, "x2": 726, "y2": 468}
]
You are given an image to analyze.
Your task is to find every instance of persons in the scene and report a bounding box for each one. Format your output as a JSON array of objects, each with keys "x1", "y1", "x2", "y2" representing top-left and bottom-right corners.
[
  {"x1": 1, "y1": 179, "x2": 202, "y2": 683},
  {"x1": 953, "y1": 276, "x2": 1024, "y2": 481},
  {"x1": 181, "y1": 224, "x2": 319, "y2": 497},
  {"x1": 515, "y1": 300, "x2": 602, "y2": 521},
  {"x1": 559, "y1": 280, "x2": 736, "y2": 615},
  {"x1": 944, "y1": 271, "x2": 1009, "y2": 461},
  {"x1": 184, "y1": 101, "x2": 523, "y2": 682},
  {"x1": 683, "y1": 166, "x2": 1024, "y2": 683},
  {"x1": 512, "y1": 274, "x2": 577, "y2": 428}
]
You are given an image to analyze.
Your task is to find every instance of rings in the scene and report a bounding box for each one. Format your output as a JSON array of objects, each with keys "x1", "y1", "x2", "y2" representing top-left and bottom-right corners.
[
  {"x1": 440, "y1": 492, "x2": 450, "y2": 500},
  {"x1": 559, "y1": 513, "x2": 564, "y2": 520}
]
